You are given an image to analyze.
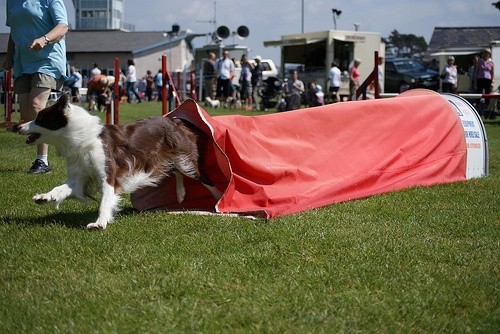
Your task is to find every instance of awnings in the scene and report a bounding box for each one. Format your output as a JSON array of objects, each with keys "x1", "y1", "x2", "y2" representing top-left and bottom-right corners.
[{"x1": 429, "y1": 47, "x2": 486, "y2": 57}]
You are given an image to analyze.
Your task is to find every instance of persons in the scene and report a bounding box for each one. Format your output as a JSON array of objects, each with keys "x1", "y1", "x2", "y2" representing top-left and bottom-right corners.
[
  {"x1": 328, "y1": 61, "x2": 341, "y2": 103},
  {"x1": 144, "y1": 70, "x2": 154, "y2": 102},
  {"x1": 118, "y1": 68, "x2": 127, "y2": 104},
  {"x1": 123, "y1": 60, "x2": 143, "y2": 104},
  {"x1": 3, "y1": 0, "x2": 69, "y2": 175},
  {"x1": 203, "y1": 50, "x2": 265, "y2": 111},
  {"x1": 70, "y1": 68, "x2": 89, "y2": 103},
  {"x1": 440, "y1": 55, "x2": 458, "y2": 95},
  {"x1": 87, "y1": 62, "x2": 115, "y2": 113},
  {"x1": 469, "y1": 48, "x2": 494, "y2": 116},
  {"x1": 427, "y1": 58, "x2": 439, "y2": 72},
  {"x1": 154, "y1": 70, "x2": 163, "y2": 102},
  {"x1": 349, "y1": 59, "x2": 361, "y2": 101},
  {"x1": 283, "y1": 70, "x2": 325, "y2": 111}
]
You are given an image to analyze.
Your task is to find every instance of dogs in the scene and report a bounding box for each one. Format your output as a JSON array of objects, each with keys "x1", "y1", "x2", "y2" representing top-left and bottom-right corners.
[{"x1": 13, "y1": 93, "x2": 222, "y2": 231}]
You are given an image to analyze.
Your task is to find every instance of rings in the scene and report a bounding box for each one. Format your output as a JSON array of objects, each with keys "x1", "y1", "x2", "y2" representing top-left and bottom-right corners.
[{"x1": 33, "y1": 46, "x2": 37, "y2": 49}]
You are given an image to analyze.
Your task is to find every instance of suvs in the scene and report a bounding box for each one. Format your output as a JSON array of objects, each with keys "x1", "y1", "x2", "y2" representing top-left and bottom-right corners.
[
  {"x1": 230, "y1": 57, "x2": 278, "y2": 99},
  {"x1": 384, "y1": 57, "x2": 440, "y2": 94}
]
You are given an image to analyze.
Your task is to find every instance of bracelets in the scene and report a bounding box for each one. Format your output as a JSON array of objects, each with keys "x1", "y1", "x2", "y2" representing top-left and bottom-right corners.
[{"x1": 43, "y1": 35, "x2": 50, "y2": 46}]
